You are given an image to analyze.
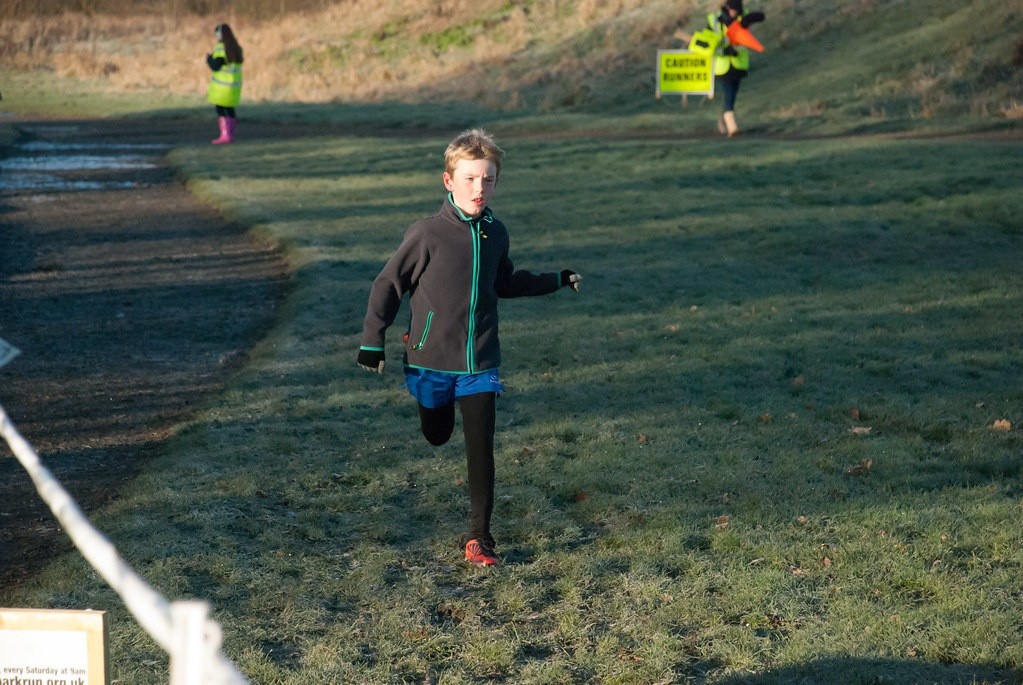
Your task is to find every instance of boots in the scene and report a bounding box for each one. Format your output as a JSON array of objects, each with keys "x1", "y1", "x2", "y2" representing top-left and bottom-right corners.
[
  {"x1": 211, "y1": 116, "x2": 236, "y2": 143},
  {"x1": 718, "y1": 108, "x2": 741, "y2": 138}
]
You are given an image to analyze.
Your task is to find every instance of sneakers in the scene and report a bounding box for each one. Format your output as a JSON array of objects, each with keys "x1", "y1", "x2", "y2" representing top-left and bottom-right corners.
[{"x1": 466, "y1": 540, "x2": 498, "y2": 566}]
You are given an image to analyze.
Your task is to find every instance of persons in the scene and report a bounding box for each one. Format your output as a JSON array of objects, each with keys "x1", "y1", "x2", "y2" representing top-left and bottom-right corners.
[
  {"x1": 206, "y1": 24, "x2": 243, "y2": 143},
  {"x1": 708, "y1": 0, "x2": 765, "y2": 136},
  {"x1": 358, "y1": 130, "x2": 583, "y2": 564}
]
